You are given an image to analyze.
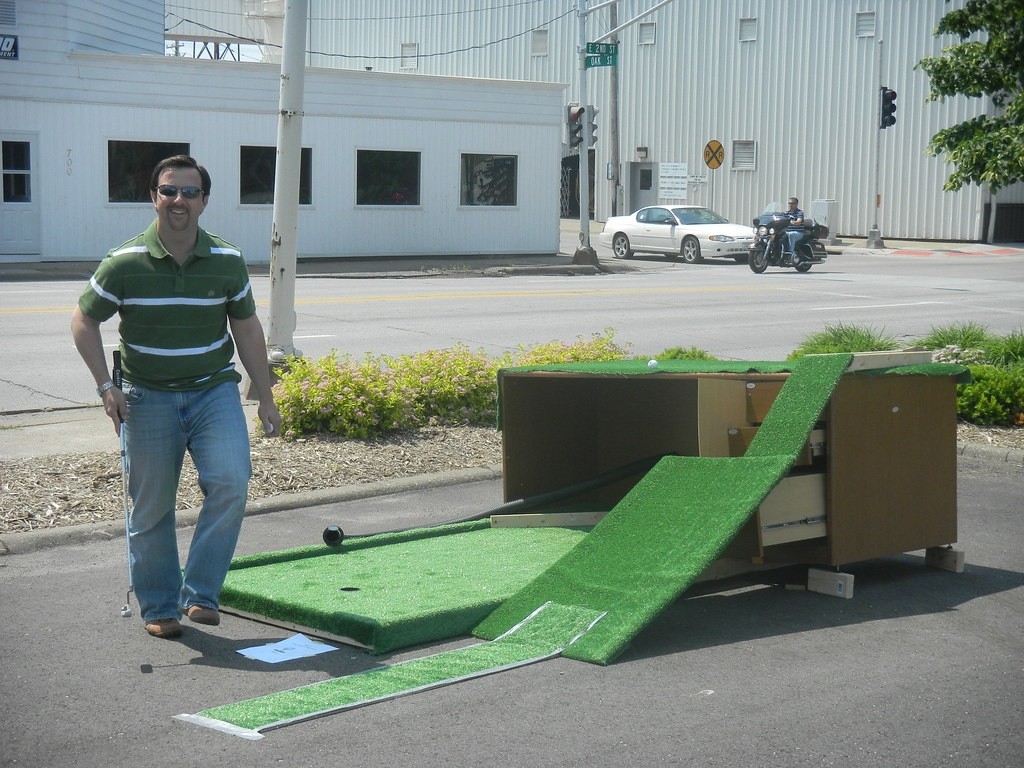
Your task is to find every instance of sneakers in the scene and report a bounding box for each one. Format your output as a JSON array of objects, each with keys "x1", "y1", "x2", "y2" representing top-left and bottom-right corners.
[
  {"x1": 178, "y1": 604, "x2": 220, "y2": 626},
  {"x1": 144, "y1": 617, "x2": 180, "y2": 637}
]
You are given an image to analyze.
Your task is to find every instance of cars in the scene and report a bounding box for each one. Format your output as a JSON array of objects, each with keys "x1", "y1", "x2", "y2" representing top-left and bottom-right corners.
[{"x1": 596, "y1": 204, "x2": 759, "y2": 264}]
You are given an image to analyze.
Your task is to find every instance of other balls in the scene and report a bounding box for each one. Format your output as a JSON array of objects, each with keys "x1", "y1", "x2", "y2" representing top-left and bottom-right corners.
[{"x1": 262, "y1": 422, "x2": 274, "y2": 433}]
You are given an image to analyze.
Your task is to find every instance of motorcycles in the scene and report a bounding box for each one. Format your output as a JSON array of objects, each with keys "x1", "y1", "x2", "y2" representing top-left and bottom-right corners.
[{"x1": 747, "y1": 202, "x2": 830, "y2": 274}]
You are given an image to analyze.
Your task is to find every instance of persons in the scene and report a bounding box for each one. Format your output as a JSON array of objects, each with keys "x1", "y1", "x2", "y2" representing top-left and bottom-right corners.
[
  {"x1": 71, "y1": 155, "x2": 282, "y2": 638},
  {"x1": 784, "y1": 198, "x2": 804, "y2": 253}
]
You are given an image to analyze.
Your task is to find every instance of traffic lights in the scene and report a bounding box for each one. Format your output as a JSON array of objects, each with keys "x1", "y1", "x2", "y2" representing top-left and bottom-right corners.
[
  {"x1": 566, "y1": 102, "x2": 585, "y2": 148},
  {"x1": 588, "y1": 104, "x2": 600, "y2": 147},
  {"x1": 880, "y1": 86, "x2": 898, "y2": 128}
]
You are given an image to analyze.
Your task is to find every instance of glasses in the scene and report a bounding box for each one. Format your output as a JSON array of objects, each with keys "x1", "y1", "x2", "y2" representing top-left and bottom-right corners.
[
  {"x1": 788, "y1": 202, "x2": 796, "y2": 205},
  {"x1": 157, "y1": 184, "x2": 205, "y2": 200}
]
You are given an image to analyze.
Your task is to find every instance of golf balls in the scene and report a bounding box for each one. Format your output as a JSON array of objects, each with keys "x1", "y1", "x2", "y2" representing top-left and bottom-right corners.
[{"x1": 647, "y1": 359, "x2": 658, "y2": 370}]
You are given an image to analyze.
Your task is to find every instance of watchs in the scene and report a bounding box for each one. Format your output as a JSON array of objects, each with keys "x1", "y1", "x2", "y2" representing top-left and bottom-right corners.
[{"x1": 96, "y1": 381, "x2": 114, "y2": 398}]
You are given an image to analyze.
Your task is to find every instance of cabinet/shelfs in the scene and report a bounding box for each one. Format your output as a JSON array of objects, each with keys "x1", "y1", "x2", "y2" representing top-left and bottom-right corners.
[{"x1": 496, "y1": 358, "x2": 975, "y2": 566}]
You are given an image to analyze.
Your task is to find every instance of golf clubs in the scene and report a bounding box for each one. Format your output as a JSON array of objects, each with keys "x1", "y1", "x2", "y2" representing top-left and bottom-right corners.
[{"x1": 112, "y1": 350, "x2": 136, "y2": 619}]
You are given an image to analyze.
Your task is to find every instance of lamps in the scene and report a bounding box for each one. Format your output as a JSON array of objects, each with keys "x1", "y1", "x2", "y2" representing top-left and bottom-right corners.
[{"x1": 636, "y1": 147, "x2": 648, "y2": 158}]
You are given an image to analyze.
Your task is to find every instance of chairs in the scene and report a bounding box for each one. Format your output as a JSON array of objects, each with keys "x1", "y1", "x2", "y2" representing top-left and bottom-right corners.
[
  {"x1": 657, "y1": 214, "x2": 667, "y2": 221},
  {"x1": 692, "y1": 211, "x2": 701, "y2": 220}
]
